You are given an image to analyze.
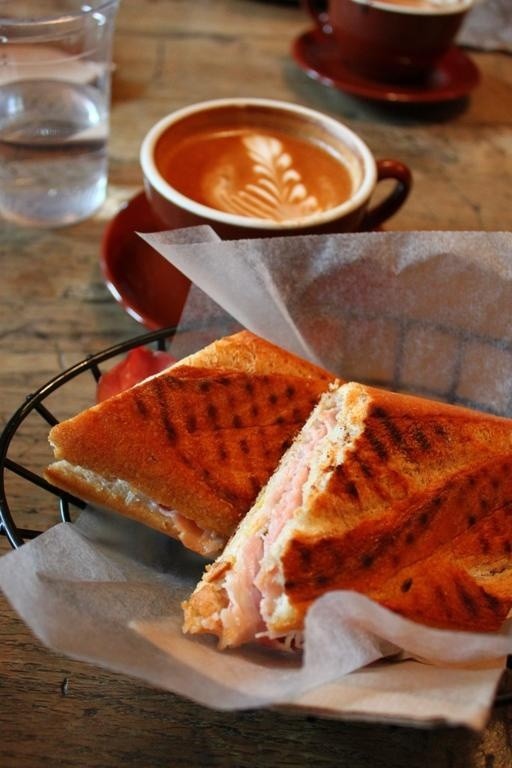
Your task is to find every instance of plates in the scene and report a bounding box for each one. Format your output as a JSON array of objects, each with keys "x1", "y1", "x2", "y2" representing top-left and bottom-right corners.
[
  {"x1": 99, "y1": 183, "x2": 387, "y2": 349},
  {"x1": 292, "y1": 23, "x2": 481, "y2": 103}
]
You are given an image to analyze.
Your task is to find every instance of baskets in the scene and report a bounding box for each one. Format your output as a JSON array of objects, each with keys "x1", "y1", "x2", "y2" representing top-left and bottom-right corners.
[{"x1": 0, "y1": 314, "x2": 512, "y2": 553}]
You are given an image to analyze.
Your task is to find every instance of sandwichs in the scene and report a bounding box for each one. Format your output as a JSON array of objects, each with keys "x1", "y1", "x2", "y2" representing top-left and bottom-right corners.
[
  {"x1": 182, "y1": 380, "x2": 512, "y2": 650},
  {"x1": 45, "y1": 329, "x2": 347, "y2": 561}
]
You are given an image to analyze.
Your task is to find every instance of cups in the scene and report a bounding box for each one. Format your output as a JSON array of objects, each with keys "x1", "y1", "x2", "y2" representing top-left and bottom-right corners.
[
  {"x1": 0, "y1": 0, "x2": 119, "y2": 230},
  {"x1": 138, "y1": 97, "x2": 414, "y2": 243},
  {"x1": 304, "y1": 0, "x2": 471, "y2": 83}
]
